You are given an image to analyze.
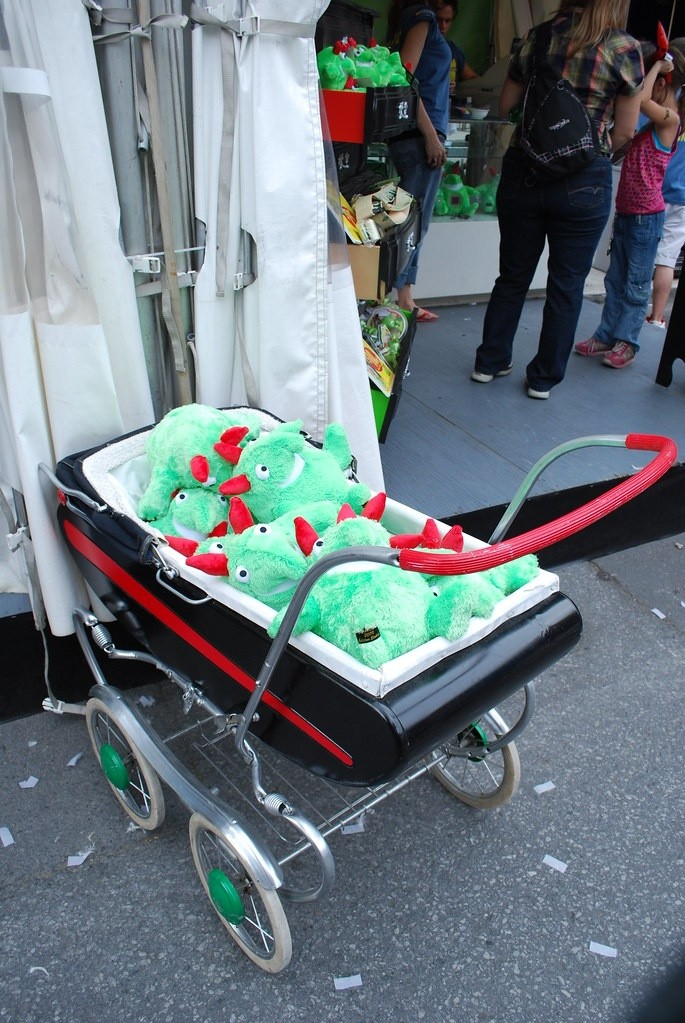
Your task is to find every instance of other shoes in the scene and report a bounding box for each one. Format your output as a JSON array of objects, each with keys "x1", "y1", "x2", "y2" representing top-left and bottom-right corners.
[{"x1": 646, "y1": 313, "x2": 666, "y2": 328}]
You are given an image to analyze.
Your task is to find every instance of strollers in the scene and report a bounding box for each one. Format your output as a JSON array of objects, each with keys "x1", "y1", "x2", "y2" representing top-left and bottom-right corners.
[{"x1": 39, "y1": 404, "x2": 679, "y2": 975}]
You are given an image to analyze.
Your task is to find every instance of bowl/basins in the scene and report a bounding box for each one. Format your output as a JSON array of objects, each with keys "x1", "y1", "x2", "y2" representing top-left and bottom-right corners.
[{"x1": 467, "y1": 108, "x2": 490, "y2": 120}]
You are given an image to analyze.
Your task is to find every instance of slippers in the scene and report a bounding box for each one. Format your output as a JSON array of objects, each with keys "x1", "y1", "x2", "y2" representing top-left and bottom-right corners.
[{"x1": 415, "y1": 309, "x2": 437, "y2": 322}]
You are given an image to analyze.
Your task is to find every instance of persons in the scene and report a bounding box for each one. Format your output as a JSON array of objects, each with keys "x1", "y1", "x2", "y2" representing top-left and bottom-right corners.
[
  {"x1": 470, "y1": 0, "x2": 645, "y2": 400},
  {"x1": 574, "y1": 37, "x2": 685, "y2": 368},
  {"x1": 377, "y1": 0, "x2": 478, "y2": 324}
]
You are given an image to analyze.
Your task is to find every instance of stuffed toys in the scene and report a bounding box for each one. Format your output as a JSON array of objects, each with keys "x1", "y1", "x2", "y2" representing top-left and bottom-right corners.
[
  {"x1": 435, "y1": 160, "x2": 502, "y2": 220},
  {"x1": 316, "y1": 37, "x2": 409, "y2": 91},
  {"x1": 135, "y1": 404, "x2": 540, "y2": 670}
]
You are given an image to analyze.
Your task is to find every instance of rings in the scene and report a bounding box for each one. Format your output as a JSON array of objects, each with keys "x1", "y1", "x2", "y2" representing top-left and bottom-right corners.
[{"x1": 433, "y1": 158, "x2": 437, "y2": 162}]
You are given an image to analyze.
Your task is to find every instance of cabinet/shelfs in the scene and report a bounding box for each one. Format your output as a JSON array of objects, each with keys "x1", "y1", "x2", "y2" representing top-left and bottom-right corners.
[{"x1": 318, "y1": 86, "x2": 423, "y2": 443}]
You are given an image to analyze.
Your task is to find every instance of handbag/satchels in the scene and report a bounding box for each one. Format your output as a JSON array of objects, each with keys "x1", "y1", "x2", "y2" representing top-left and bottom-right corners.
[{"x1": 516, "y1": 22, "x2": 610, "y2": 174}]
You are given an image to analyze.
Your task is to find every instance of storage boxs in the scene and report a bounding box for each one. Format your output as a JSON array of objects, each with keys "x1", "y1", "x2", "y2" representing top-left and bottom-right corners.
[
  {"x1": 323, "y1": 89, "x2": 370, "y2": 145},
  {"x1": 342, "y1": 243, "x2": 382, "y2": 302},
  {"x1": 363, "y1": 339, "x2": 397, "y2": 436}
]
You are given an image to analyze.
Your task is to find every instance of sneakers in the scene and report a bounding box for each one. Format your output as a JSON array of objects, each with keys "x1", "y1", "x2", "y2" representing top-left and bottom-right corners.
[
  {"x1": 575, "y1": 335, "x2": 611, "y2": 357},
  {"x1": 526, "y1": 380, "x2": 552, "y2": 398},
  {"x1": 472, "y1": 360, "x2": 513, "y2": 383},
  {"x1": 603, "y1": 340, "x2": 635, "y2": 368}
]
